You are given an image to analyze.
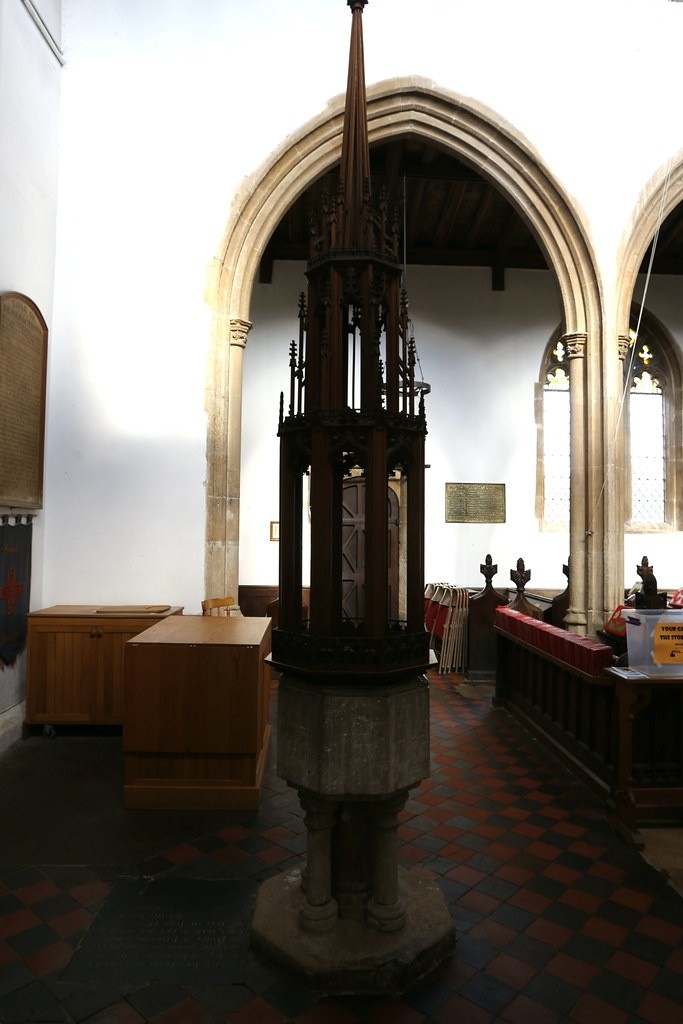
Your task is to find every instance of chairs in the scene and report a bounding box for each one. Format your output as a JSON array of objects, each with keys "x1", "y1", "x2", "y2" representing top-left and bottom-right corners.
[{"x1": 424, "y1": 583, "x2": 469, "y2": 675}]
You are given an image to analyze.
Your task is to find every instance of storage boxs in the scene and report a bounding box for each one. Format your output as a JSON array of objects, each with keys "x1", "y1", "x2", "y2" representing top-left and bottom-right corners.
[{"x1": 621, "y1": 608, "x2": 683, "y2": 677}]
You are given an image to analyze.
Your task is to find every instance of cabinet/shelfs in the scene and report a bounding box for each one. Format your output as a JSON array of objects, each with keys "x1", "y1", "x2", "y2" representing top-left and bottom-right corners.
[
  {"x1": 26, "y1": 617, "x2": 182, "y2": 736},
  {"x1": 125, "y1": 614, "x2": 272, "y2": 810}
]
